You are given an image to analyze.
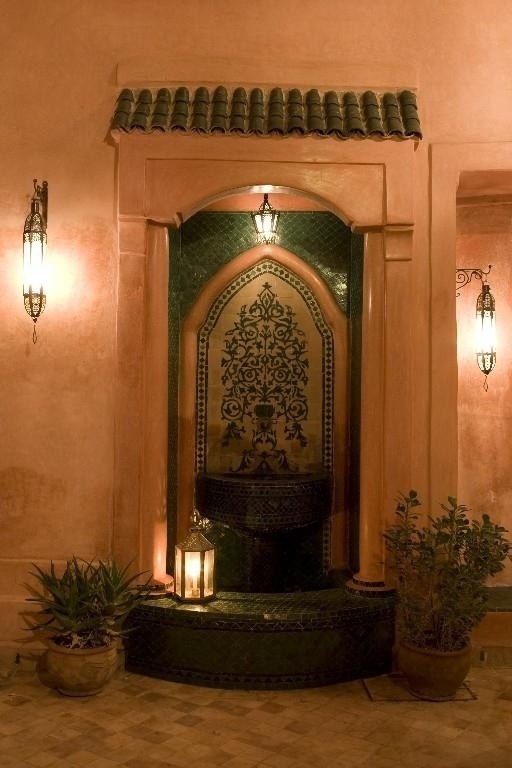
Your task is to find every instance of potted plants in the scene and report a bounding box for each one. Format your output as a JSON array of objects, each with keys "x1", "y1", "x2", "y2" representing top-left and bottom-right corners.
[
  {"x1": 376, "y1": 492, "x2": 509, "y2": 698},
  {"x1": 17, "y1": 550, "x2": 157, "y2": 698}
]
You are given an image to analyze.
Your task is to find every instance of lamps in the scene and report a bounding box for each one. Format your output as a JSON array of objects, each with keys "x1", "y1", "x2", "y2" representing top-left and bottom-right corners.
[
  {"x1": 16, "y1": 181, "x2": 54, "y2": 330},
  {"x1": 173, "y1": 512, "x2": 220, "y2": 603},
  {"x1": 249, "y1": 192, "x2": 281, "y2": 247},
  {"x1": 454, "y1": 265, "x2": 498, "y2": 394}
]
